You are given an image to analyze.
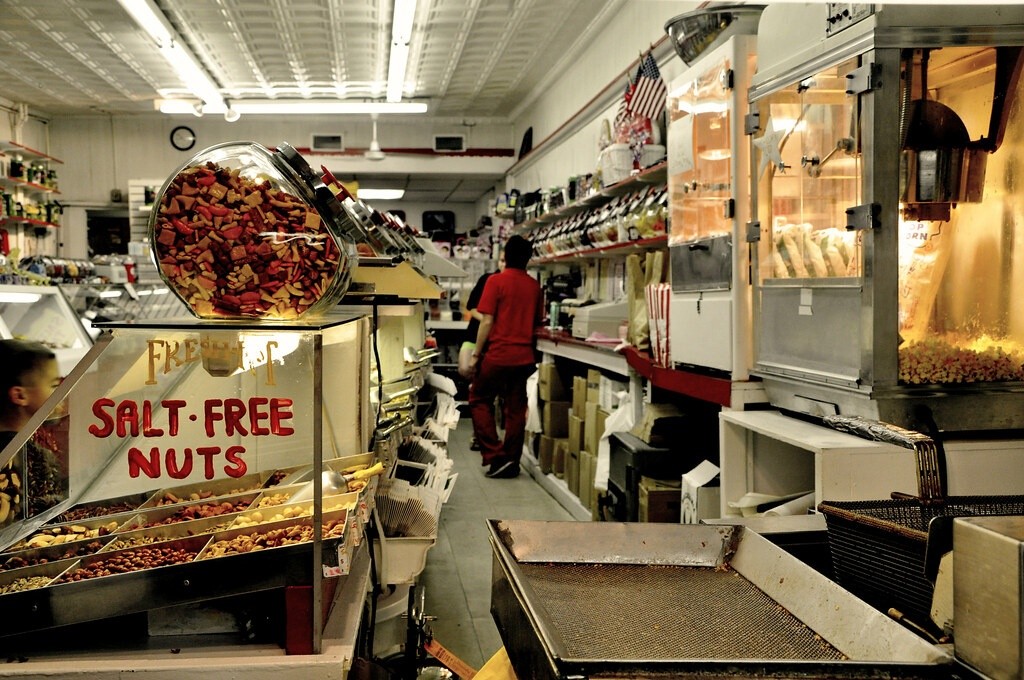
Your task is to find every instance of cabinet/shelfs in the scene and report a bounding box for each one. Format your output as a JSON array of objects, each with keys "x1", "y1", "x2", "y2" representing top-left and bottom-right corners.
[
  {"x1": 508, "y1": 161, "x2": 668, "y2": 522},
  {"x1": 0, "y1": 139, "x2": 63, "y2": 230}
]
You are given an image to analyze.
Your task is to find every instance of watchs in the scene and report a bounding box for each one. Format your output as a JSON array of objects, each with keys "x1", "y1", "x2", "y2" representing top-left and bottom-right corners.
[{"x1": 470, "y1": 352, "x2": 479, "y2": 358}]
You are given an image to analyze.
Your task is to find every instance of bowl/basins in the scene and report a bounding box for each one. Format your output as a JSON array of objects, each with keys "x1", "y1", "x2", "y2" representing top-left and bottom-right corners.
[{"x1": 664, "y1": 4, "x2": 767, "y2": 68}]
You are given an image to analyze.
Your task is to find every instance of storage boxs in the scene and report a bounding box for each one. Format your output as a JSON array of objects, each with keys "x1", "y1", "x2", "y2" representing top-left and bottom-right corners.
[{"x1": 528, "y1": 353, "x2": 719, "y2": 524}]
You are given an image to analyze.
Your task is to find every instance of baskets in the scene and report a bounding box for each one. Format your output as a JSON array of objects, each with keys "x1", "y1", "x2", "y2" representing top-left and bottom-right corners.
[{"x1": 818, "y1": 496, "x2": 1024, "y2": 625}]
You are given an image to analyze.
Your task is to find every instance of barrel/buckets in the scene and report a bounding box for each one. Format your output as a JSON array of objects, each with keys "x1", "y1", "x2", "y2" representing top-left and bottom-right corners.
[
  {"x1": 638, "y1": 145, "x2": 665, "y2": 168},
  {"x1": 600, "y1": 143, "x2": 633, "y2": 187}
]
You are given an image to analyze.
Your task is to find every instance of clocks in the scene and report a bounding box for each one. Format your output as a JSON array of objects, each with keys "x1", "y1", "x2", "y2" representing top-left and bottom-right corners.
[{"x1": 170, "y1": 126, "x2": 196, "y2": 152}]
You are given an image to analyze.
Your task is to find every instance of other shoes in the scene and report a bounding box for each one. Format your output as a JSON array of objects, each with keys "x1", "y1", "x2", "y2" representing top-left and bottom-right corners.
[
  {"x1": 485, "y1": 454, "x2": 521, "y2": 478},
  {"x1": 471, "y1": 435, "x2": 480, "y2": 451}
]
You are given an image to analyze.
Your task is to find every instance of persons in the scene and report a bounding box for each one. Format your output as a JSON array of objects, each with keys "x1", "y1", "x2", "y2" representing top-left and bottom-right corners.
[
  {"x1": 0, "y1": 337, "x2": 63, "y2": 452},
  {"x1": 467, "y1": 234, "x2": 544, "y2": 478},
  {"x1": 457, "y1": 247, "x2": 507, "y2": 451}
]
